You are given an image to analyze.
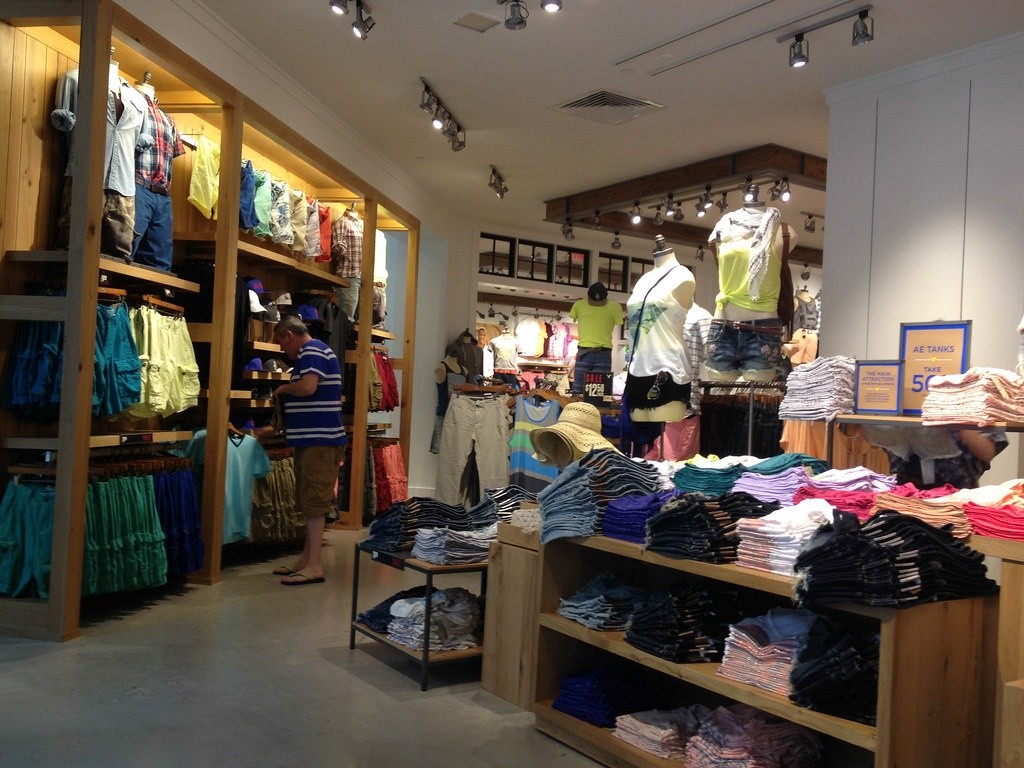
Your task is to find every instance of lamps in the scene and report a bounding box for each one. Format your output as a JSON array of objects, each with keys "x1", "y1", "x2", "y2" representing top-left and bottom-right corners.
[{"x1": 323, "y1": 0, "x2": 874, "y2": 280}]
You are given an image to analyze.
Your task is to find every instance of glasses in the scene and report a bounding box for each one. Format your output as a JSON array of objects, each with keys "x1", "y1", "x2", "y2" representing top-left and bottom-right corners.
[{"x1": 646, "y1": 369, "x2": 668, "y2": 400}]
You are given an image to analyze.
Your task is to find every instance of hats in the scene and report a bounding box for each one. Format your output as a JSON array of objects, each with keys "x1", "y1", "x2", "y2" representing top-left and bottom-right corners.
[
  {"x1": 587, "y1": 282, "x2": 608, "y2": 301},
  {"x1": 528, "y1": 401, "x2": 615, "y2": 472}
]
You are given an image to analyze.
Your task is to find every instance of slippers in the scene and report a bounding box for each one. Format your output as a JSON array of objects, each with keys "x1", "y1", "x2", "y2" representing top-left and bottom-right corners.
[
  {"x1": 272, "y1": 562, "x2": 298, "y2": 576},
  {"x1": 281, "y1": 570, "x2": 326, "y2": 586}
]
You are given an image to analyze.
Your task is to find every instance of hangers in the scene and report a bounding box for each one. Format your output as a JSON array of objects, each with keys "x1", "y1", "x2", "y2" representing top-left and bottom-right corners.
[
  {"x1": 0, "y1": 284, "x2": 399, "y2": 484},
  {"x1": 451, "y1": 367, "x2": 787, "y2": 424}
]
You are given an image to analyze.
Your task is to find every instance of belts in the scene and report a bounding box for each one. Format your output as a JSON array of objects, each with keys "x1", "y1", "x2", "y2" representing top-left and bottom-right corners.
[
  {"x1": 134, "y1": 177, "x2": 172, "y2": 197},
  {"x1": 493, "y1": 368, "x2": 517, "y2": 375},
  {"x1": 373, "y1": 281, "x2": 386, "y2": 288},
  {"x1": 577, "y1": 345, "x2": 612, "y2": 356},
  {"x1": 711, "y1": 319, "x2": 785, "y2": 336}
]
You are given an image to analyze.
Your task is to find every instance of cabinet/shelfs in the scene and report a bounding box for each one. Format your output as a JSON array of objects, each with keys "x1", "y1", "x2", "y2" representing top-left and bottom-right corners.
[
  {"x1": 0, "y1": 0, "x2": 420, "y2": 641},
  {"x1": 533, "y1": 414, "x2": 1024, "y2": 768},
  {"x1": 481, "y1": 522, "x2": 540, "y2": 712},
  {"x1": 350, "y1": 543, "x2": 488, "y2": 691}
]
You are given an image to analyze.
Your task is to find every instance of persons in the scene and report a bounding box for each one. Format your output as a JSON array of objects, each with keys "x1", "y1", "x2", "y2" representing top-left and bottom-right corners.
[
  {"x1": 567, "y1": 294, "x2": 624, "y2": 394},
  {"x1": 630, "y1": 302, "x2": 714, "y2": 461},
  {"x1": 707, "y1": 200, "x2": 798, "y2": 382},
  {"x1": 372, "y1": 229, "x2": 390, "y2": 332},
  {"x1": 889, "y1": 427, "x2": 1010, "y2": 490},
  {"x1": 488, "y1": 330, "x2": 523, "y2": 386},
  {"x1": 621, "y1": 246, "x2": 696, "y2": 423},
  {"x1": 48, "y1": 57, "x2": 154, "y2": 263},
  {"x1": 330, "y1": 208, "x2": 364, "y2": 318},
  {"x1": 131, "y1": 81, "x2": 187, "y2": 274},
  {"x1": 446, "y1": 330, "x2": 484, "y2": 385},
  {"x1": 273, "y1": 317, "x2": 349, "y2": 587},
  {"x1": 429, "y1": 354, "x2": 469, "y2": 454},
  {"x1": 791, "y1": 290, "x2": 817, "y2": 364}
]
